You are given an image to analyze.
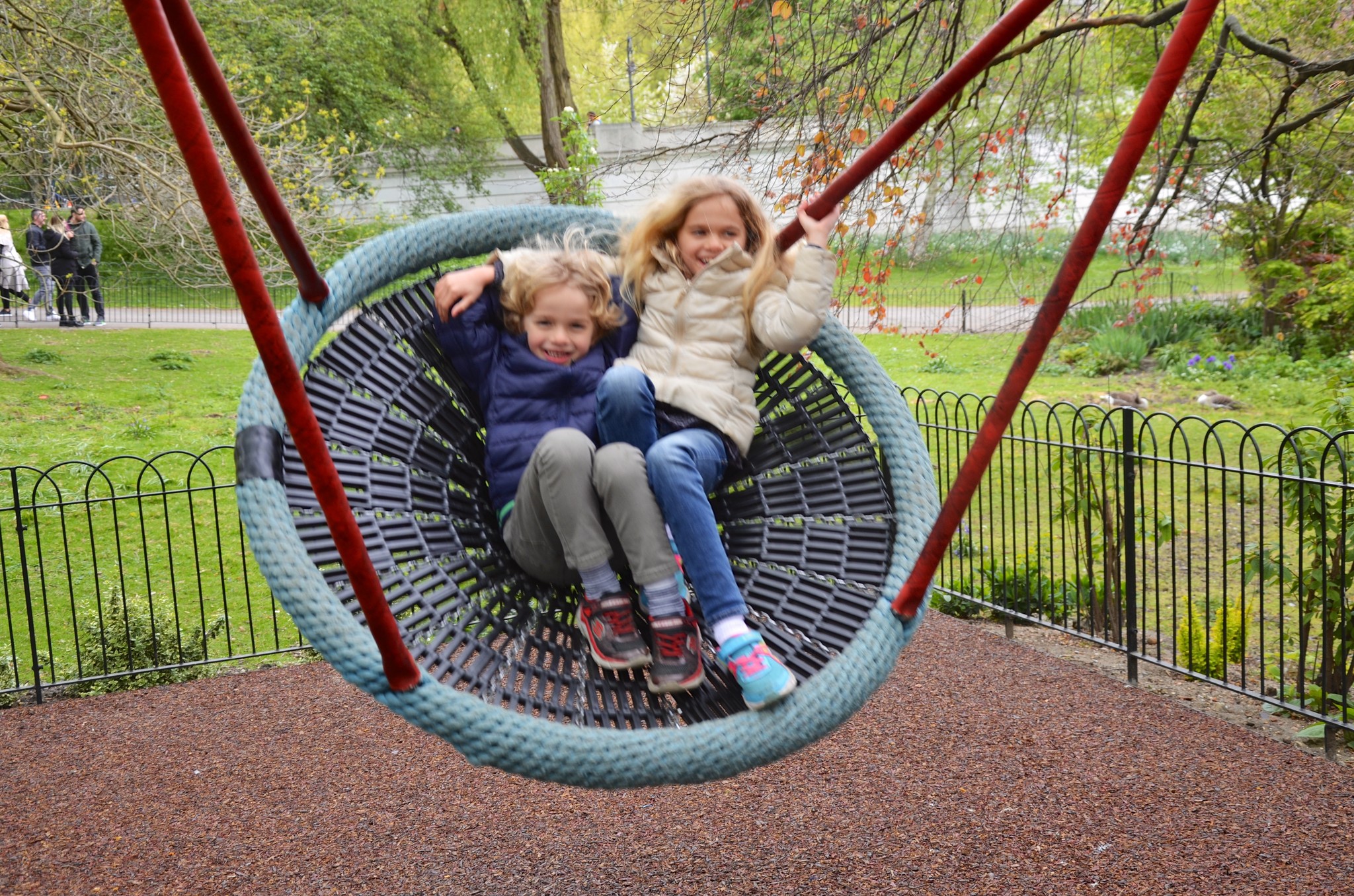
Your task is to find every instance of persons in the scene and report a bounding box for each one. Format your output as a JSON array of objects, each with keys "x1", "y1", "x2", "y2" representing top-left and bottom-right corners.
[
  {"x1": 434, "y1": 175, "x2": 848, "y2": 714},
  {"x1": 438, "y1": 224, "x2": 705, "y2": 693},
  {"x1": 0, "y1": 205, "x2": 106, "y2": 327}
]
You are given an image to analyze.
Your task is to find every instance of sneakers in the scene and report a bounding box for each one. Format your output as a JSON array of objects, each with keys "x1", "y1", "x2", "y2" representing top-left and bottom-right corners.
[
  {"x1": 641, "y1": 598, "x2": 703, "y2": 696},
  {"x1": 638, "y1": 536, "x2": 691, "y2": 615},
  {"x1": 573, "y1": 590, "x2": 650, "y2": 672},
  {"x1": 715, "y1": 627, "x2": 797, "y2": 709}
]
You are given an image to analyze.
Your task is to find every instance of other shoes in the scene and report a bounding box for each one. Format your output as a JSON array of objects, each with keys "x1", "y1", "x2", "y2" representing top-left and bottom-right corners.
[
  {"x1": 0, "y1": 308, "x2": 12, "y2": 317},
  {"x1": 59, "y1": 315, "x2": 73, "y2": 327},
  {"x1": 79, "y1": 315, "x2": 91, "y2": 326},
  {"x1": 93, "y1": 315, "x2": 106, "y2": 326},
  {"x1": 67, "y1": 317, "x2": 84, "y2": 327},
  {"x1": 22, "y1": 309, "x2": 35, "y2": 321},
  {"x1": 45, "y1": 310, "x2": 61, "y2": 322}
]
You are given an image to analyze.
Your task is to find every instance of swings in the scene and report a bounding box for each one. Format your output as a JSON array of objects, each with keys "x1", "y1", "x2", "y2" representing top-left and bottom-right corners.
[{"x1": 123, "y1": 0, "x2": 1222, "y2": 792}]
